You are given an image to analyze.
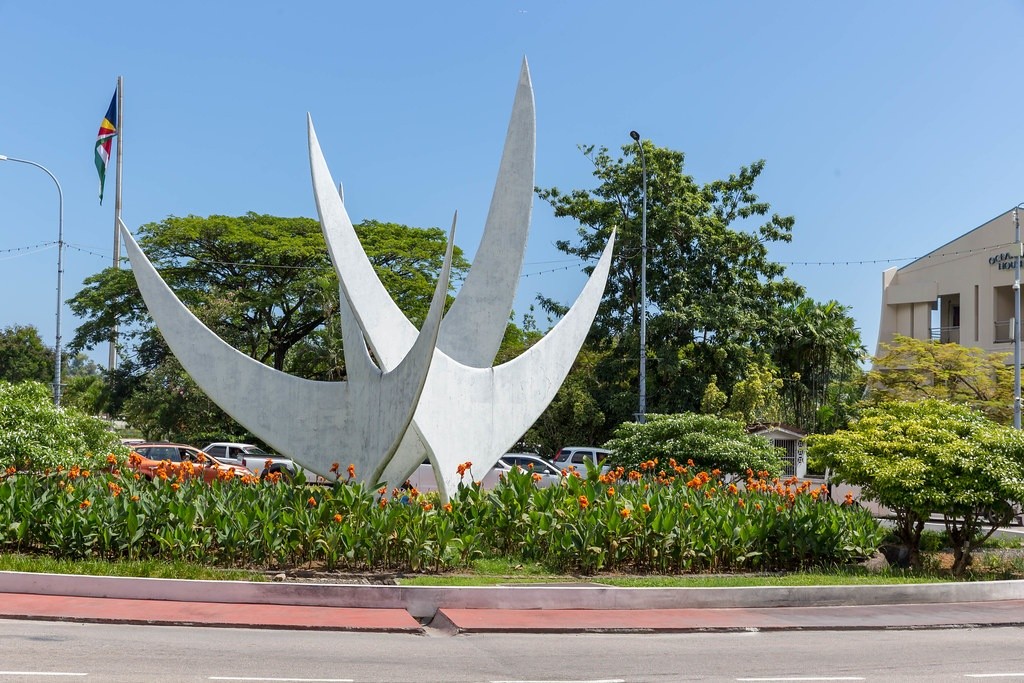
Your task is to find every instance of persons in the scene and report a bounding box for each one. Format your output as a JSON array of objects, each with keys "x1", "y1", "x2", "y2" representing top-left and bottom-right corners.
[
  {"x1": 231, "y1": 449, "x2": 242, "y2": 458},
  {"x1": 180, "y1": 452, "x2": 199, "y2": 462}
]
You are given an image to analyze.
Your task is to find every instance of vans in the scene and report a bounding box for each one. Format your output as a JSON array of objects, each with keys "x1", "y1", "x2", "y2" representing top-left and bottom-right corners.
[
  {"x1": 553, "y1": 445, "x2": 616, "y2": 477},
  {"x1": 500, "y1": 453, "x2": 568, "y2": 489}
]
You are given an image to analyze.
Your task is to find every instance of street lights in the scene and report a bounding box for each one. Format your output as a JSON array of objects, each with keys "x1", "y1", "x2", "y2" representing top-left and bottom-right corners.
[
  {"x1": 627, "y1": 124, "x2": 660, "y2": 426},
  {"x1": 0, "y1": 154, "x2": 67, "y2": 408}
]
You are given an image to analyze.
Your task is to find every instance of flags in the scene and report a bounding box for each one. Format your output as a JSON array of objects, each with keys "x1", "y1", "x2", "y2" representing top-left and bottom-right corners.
[{"x1": 94, "y1": 85, "x2": 118, "y2": 206}]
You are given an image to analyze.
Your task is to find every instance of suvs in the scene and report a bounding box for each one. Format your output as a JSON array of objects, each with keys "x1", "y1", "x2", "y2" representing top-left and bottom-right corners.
[
  {"x1": 204, "y1": 439, "x2": 303, "y2": 478},
  {"x1": 130, "y1": 439, "x2": 256, "y2": 486}
]
